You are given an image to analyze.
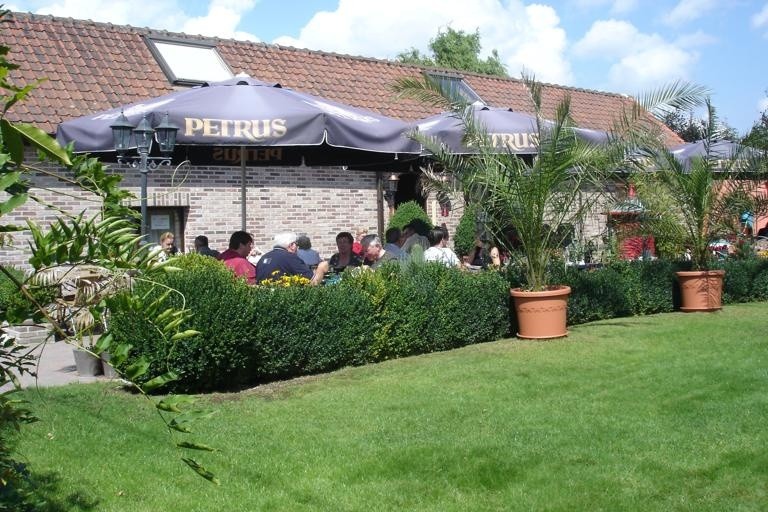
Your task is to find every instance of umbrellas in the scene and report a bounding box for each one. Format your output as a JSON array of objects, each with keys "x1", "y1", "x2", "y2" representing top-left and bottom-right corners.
[
  {"x1": 523, "y1": 124, "x2": 673, "y2": 244},
  {"x1": 56, "y1": 71, "x2": 424, "y2": 233},
  {"x1": 656, "y1": 138, "x2": 768, "y2": 180},
  {"x1": 346, "y1": 100, "x2": 616, "y2": 173}
]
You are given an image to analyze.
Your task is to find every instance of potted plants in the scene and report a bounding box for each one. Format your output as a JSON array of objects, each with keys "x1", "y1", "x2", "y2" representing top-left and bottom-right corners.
[
  {"x1": 600, "y1": 89, "x2": 768, "y2": 312},
  {"x1": 388, "y1": 66, "x2": 719, "y2": 342},
  {"x1": 70, "y1": 328, "x2": 102, "y2": 375},
  {"x1": 93, "y1": 323, "x2": 124, "y2": 379}
]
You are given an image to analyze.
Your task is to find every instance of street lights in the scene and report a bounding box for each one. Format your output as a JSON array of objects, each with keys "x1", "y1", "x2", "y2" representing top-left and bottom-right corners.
[{"x1": 109, "y1": 108, "x2": 179, "y2": 258}]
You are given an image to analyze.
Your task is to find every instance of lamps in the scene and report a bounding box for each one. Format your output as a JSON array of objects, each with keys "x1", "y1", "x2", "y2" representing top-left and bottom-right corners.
[{"x1": 381, "y1": 173, "x2": 400, "y2": 202}]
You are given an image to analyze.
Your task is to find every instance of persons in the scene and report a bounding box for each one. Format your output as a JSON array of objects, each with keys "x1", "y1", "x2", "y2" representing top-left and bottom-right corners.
[
  {"x1": 757, "y1": 223, "x2": 768, "y2": 241},
  {"x1": 739, "y1": 211, "x2": 754, "y2": 241}
]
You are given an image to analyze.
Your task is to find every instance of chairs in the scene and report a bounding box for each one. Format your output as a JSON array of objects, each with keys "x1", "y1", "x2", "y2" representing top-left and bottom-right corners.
[{"x1": 61, "y1": 275, "x2": 110, "y2": 344}]
